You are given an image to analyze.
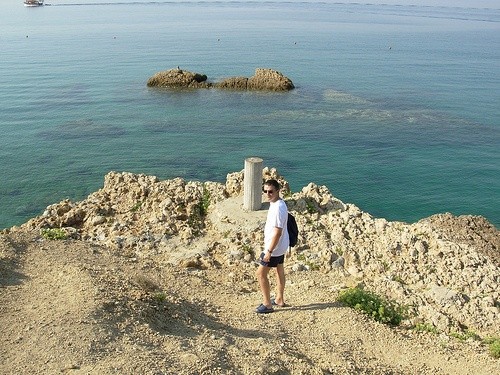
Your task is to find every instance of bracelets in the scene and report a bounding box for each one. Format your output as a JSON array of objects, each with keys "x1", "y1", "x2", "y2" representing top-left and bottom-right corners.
[{"x1": 267, "y1": 249, "x2": 272, "y2": 253}]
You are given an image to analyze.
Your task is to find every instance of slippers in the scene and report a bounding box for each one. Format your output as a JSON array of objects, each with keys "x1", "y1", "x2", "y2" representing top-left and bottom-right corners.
[
  {"x1": 271, "y1": 299, "x2": 285, "y2": 307},
  {"x1": 255, "y1": 304, "x2": 274, "y2": 314}
]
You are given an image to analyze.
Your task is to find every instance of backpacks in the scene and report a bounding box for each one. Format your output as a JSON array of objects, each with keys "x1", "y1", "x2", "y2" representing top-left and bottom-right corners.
[{"x1": 286, "y1": 213, "x2": 299, "y2": 247}]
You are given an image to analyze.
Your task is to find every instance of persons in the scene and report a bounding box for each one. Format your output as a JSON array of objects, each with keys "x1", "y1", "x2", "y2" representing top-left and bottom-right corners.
[{"x1": 253, "y1": 180, "x2": 290, "y2": 312}]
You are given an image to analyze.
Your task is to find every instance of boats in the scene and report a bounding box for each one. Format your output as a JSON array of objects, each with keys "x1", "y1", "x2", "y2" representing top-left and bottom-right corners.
[{"x1": 24, "y1": 0, "x2": 44, "y2": 7}]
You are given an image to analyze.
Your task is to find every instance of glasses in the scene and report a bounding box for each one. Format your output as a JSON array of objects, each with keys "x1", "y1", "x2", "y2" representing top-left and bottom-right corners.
[{"x1": 263, "y1": 189, "x2": 277, "y2": 194}]
